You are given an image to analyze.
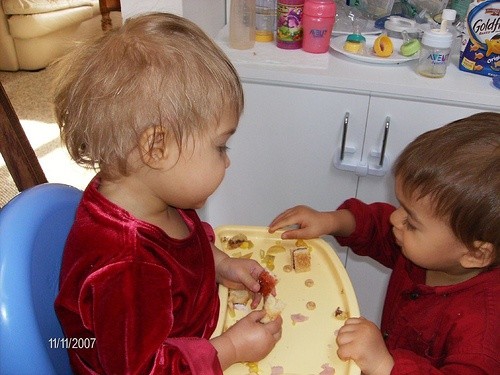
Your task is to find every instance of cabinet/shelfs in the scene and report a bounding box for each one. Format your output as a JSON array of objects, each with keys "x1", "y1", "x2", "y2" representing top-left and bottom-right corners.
[{"x1": 195, "y1": 26, "x2": 500, "y2": 328}]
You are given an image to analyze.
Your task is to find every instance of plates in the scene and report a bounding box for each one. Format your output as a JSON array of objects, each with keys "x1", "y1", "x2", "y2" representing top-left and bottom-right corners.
[{"x1": 329, "y1": 34, "x2": 420, "y2": 65}]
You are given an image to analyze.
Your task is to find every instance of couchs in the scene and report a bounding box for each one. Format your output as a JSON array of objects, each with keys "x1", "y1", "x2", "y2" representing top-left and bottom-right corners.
[{"x1": 0, "y1": 0, "x2": 121, "y2": 73}]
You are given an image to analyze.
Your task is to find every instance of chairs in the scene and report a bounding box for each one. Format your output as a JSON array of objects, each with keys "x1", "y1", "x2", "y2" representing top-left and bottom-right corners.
[{"x1": 0, "y1": 183, "x2": 363, "y2": 375}]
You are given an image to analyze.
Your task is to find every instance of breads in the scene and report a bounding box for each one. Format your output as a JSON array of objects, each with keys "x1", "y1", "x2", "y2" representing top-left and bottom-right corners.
[{"x1": 221, "y1": 234, "x2": 312, "y2": 322}]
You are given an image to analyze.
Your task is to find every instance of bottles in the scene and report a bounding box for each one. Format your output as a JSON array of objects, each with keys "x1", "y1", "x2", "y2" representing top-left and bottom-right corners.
[
  {"x1": 275, "y1": 0, "x2": 305, "y2": 49},
  {"x1": 229, "y1": 0, "x2": 256, "y2": 50},
  {"x1": 301, "y1": 0, "x2": 336, "y2": 54},
  {"x1": 415, "y1": 29, "x2": 452, "y2": 78},
  {"x1": 253, "y1": 0, "x2": 276, "y2": 42}
]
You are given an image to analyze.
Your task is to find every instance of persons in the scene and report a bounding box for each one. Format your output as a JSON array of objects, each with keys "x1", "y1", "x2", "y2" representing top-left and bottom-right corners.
[
  {"x1": 269, "y1": 111, "x2": 500, "y2": 375},
  {"x1": 51, "y1": 12, "x2": 283, "y2": 375}
]
